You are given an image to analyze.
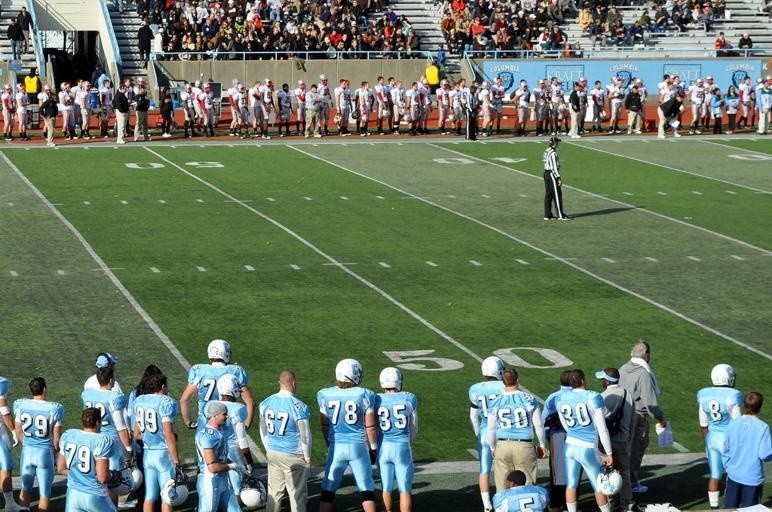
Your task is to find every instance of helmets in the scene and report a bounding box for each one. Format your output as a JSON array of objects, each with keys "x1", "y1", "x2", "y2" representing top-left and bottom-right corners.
[
  {"x1": 160, "y1": 480, "x2": 189, "y2": 507},
  {"x1": 379, "y1": 367, "x2": 403, "y2": 391},
  {"x1": 334, "y1": 358, "x2": 362, "y2": 385},
  {"x1": 239, "y1": 476, "x2": 267, "y2": 508},
  {"x1": 216, "y1": 373, "x2": 240, "y2": 399},
  {"x1": 206, "y1": 338, "x2": 231, "y2": 363},
  {"x1": 481, "y1": 356, "x2": 505, "y2": 380},
  {"x1": 710, "y1": 363, "x2": 735, "y2": 387},
  {"x1": 595, "y1": 464, "x2": 623, "y2": 495},
  {"x1": 131, "y1": 467, "x2": 143, "y2": 490},
  {"x1": 112, "y1": 466, "x2": 134, "y2": 496}
]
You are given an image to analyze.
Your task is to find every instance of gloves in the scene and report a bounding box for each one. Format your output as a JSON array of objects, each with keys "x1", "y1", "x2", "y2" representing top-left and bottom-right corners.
[
  {"x1": 125, "y1": 451, "x2": 137, "y2": 467},
  {"x1": 172, "y1": 464, "x2": 186, "y2": 482},
  {"x1": 369, "y1": 448, "x2": 377, "y2": 465},
  {"x1": 11, "y1": 429, "x2": 18, "y2": 447}
]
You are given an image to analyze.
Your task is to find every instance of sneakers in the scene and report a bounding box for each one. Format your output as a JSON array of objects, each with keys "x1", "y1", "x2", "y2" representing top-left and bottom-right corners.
[
  {"x1": 632, "y1": 485, "x2": 648, "y2": 493},
  {"x1": 118, "y1": 501, "x2": 136, "y2": 508},
  {"x1": 4, "y1": 503, "x2": 31, "y2": 512},
  {"x1": 557, "y1": 215, "x2": 571, "y2": 220},
  {"x1": 128, "y1": 498, "x2": 138, "y2": 504},
  {"x1": 543, "y1": 216, "x2": 557, "y2": 220}
]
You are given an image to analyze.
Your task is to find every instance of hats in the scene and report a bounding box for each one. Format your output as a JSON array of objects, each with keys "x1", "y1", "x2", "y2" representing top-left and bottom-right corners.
[
  {"x1": 595, "y1": 367, "x2": 620, "y2": 383},
  {"x1": 206, "y1": 403, "x2": 228, "y2": 417},
  {"x1": 96, "y1": 353, "x2": 117, "y2": 368},
  {"x1": 549, "y1": 137, "x2": 561, "y2": 144}
]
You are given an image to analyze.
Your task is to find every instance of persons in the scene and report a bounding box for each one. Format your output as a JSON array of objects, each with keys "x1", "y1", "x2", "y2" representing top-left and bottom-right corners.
[
  {"x1": 180, "y1": 339, "x2": 256, "y2": 431},
  {"x1": 182, "y1": 85, "x2": 201, "y2": 138},
  {"x1": 740, "y1": 33, "x2": 753, "y2": 57},
  {"x1": 697, "y1": 364, "x2": 746, "y2": 509},
  {"x1": 117, "y1": 77, "x2": 136, "y2": 135},
  {"x1": 486, "y1": 368, "x2": 547, "y2": 492},
  {"x1": 58, "y1": 407, "x2": 119, "y2": 512},
  {"x1": 158, "y1": 87, "x2": 175, "y2": 138},
  {"x1": 16, "y1": 83, "x2": 31, "y2": 141},
  {"x1": 80, "y1": 81, "x2": 96, "y2": 140},
  {"x1": 134, "y1": 77, "x2": 148, "y2": 100},
  {"x1": 542, "y1": 137, "x2": 572, "y2": 221},
  {"x1": 763, "y1": 0, "x2": 772, "y2": 20},
  {"x1": 58, "y1": 82, "x2": 79, "y2": 141},
  {"x1": 203, "y1": 374, "x2": 254, "y2": 506},
  {"x1": 94, "y1": 70, "x2": 111, "y2": 90},
  {"x1": 133, "y1": 87, "x2": 152, "y2": 142},
  {"x1": 137, "y1": 0, "x2": 420, "y2": 58},
  {"x1": 618, "y1": 342, "x2": 666, "y2": 494},
  {"x1": 316, "y1": 358, "x2": 378, "y2": 512},
  {"x1": 134, "y1": 373, "x2": 179, "y2": 512},
  {"x1": 433, "y1": 0, "x2": 580, "y2": 60},
  {"x1": 17, "y1": 7, "x2": 34, "y2": 54},
  {"x1": 492, "y1": 471, "x2": 550, "y2": 512},
  {"x1": 229, "y1": 72, "x2": 334, "y2": 140},
  {"x1": 196, "y1": 84, "x2": 217, "y2": 137},
  {"x1": 558, "y1": 369, "x2": 614, "y2": 512},
  {"x1": 335, "y1": 61, "x2": 505, "y2": 140},
  {"x1": 91, "y1": 65, "x2": 105, "y2": 87},
  {"x1": 39, "y1": 93, "x2": 58, "y2": 146},
  {"x1": 541, "y1": 371, "x2": 567, "y2": 510},
  {"x1": 71, "y1": 79, "x2": 86, "y2": 137},
  {"x1": 0, "y1": 377, "x2": 30, "y2": 512},
  {"x1": 509, "y1": 74, "x2": 772, "y2": 137},
  {"x1": 112, "y1": 0, "x2": 133, "y2": 15},
  {"x1": 113, "y1": 82, "x2": 129, "y2": 144},
  {"x1": 1, "y1": 83, "x2": 14, "y2": 141},
  {"x1": 7, "y1": 18, "x2": 25, "y2": 61},
  {"x1": 24, "y1": 67, "x2": 42, "y2": 104},
  {"x1": 258, "y1": 369, "x2": 312, "y2": 512},
  {"x1": 81, "y1": 367, "x2": 138, "y2": 508},
  {"x1": 594, "y1": 367, "x2": 636, "y2": 512},
  {"x1": 584, "y1": 1, "x2": 725, "y2": 48},
  {"x1": 38, "y1": 84, "x2": 51, "y2": 138},
  {"x1": 100, "y1": 80, "x2": 113, "y2": 139},
  {"x1": 721, "y1": 391, "x2": 772, "y2": 509},
  {"x1": 85, "y1": 353, "x2": 131, "y2": 439},
  {"x1": 128, "y1": 365, "x2": 177, "y2": 470},
  {"x1": 102, "y1": 73, "x2": 112, "y2": 90},
  {"x1": 468, "y1": 356, "x2": 506, "y2": 512},
  {"x1": 191, "y1": 80, "x2": 204, "y2": 129},
  {"x1": 194, "y1": 402, "x2": 243, "y2": 512},
  {"x1": 13, "y1": 377, "x2": 64, "y2": 512},
  {"x1": 374, "y1": 367, "x2": 418, "y2": 512}
]
